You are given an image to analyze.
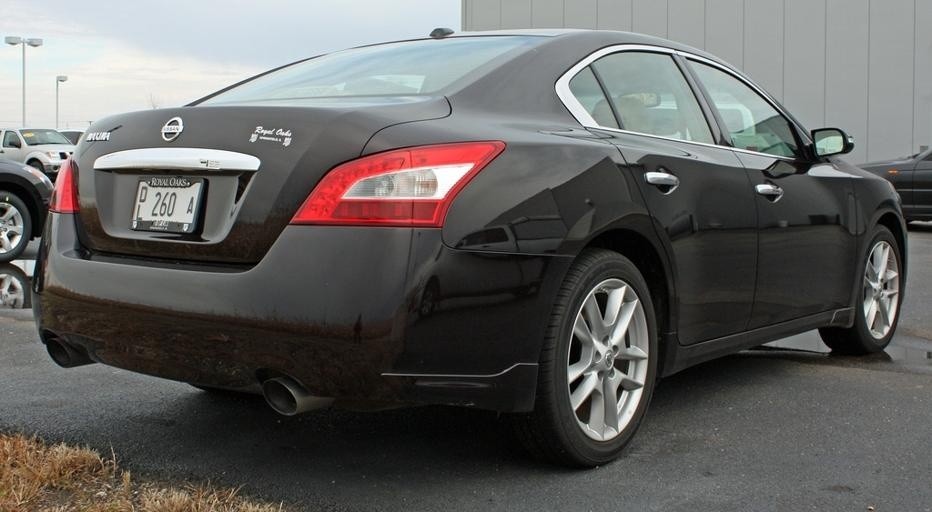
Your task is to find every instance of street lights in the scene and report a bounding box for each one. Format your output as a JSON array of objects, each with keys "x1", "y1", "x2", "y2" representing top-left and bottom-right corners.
[
  {"x1": 55, "y1": 75, "x2": 68, "y2": 128},
  {"x1": 4, "y1": 35, "x2": 44, "y2": 128}
]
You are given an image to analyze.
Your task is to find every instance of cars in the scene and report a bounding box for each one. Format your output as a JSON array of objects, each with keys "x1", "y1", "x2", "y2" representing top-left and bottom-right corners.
[
  {"x1": 0, "y1": 126, "x2": 84, "y2": 260},
  {"x1": 1, "y1": 262, "x2": 31, "y2": 310},
  {"x1": 854, "y1": 142, "x2": 930, "y2": 223},
  {"x1": 33, "y1": 27, "x2": 907, "y2": 469}
]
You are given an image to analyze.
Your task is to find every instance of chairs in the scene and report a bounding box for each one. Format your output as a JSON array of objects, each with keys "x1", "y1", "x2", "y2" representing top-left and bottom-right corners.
[{"x1": 593, "y1": 97, "x2": 657, "y2": 137}]
